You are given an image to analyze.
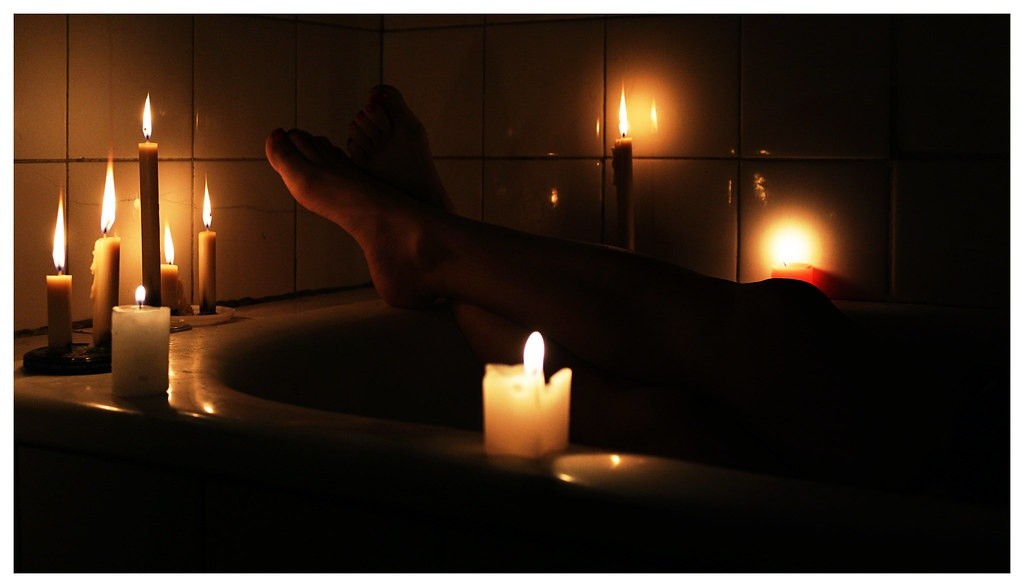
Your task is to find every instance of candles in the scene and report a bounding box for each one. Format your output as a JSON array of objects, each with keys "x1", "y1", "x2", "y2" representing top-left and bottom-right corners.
[
  {"x1": 160, "y1": 223, "x2": 181, "y2": 316},
  {"x1": 90, "y1": 145, "x2": 122, "y2": 349},
  {"x1": 767, "y1": 218, "x2": 813, "y2": 285},
  {"x1": 45, "y1": 186, "x2": 73, "y2": 353},
  {"x1": 139, "y1": 91, "x2": 160, "y2": 307},
  {"x1": 110, "y1": 285, "x2": 170, "y2": 398},
  {"x1": 198, "y1": 172, "x2": 216, "y2": 315},
  {"x1": 611, "y1": 79, "x2": 635, "y2": 251},
  {"x1": 481, "y1": 331, "x2": 572, "y2": 459}
]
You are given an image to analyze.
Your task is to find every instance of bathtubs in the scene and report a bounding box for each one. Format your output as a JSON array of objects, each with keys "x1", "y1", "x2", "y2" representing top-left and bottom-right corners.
[{"x1": 17, "y1": 299, "x2": 1010, "y2": 568}]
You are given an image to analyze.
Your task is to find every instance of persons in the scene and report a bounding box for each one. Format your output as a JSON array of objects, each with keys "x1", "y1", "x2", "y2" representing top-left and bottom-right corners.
[{"x1": 265, "y1": 83, "x2": 1010, "y2": 505}]
